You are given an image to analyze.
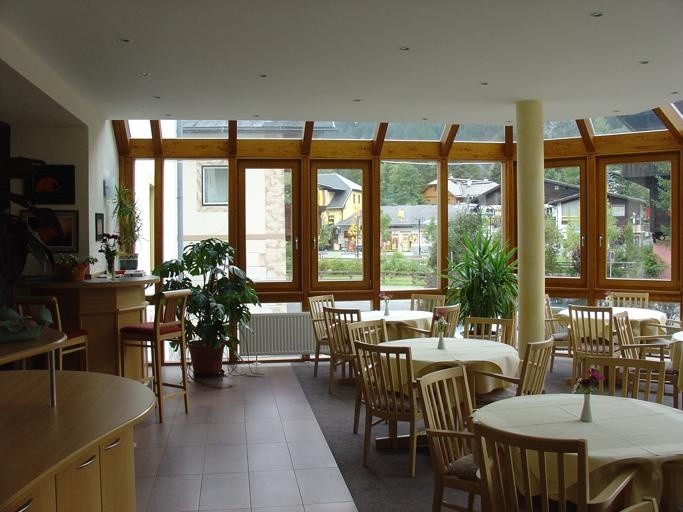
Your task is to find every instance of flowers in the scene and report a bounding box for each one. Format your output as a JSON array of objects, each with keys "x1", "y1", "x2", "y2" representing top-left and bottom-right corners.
[
  {"x1": 571, "y1": 369, "x2": 603, "y2": 395},
  {"x1": 98, "y1": 233, "x2": 121, "y2": 251},
  {"x1": 432, "y1": 312, "x2": 448, "y2": 336},
  {"x1": 378, "y1": 293, "x2": 393, "y2": 303}
]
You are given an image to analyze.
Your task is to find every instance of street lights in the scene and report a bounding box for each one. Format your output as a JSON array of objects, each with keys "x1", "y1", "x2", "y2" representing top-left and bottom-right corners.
[{"x1": 413, "y1": 216, "x2": 425, "y2": 257}]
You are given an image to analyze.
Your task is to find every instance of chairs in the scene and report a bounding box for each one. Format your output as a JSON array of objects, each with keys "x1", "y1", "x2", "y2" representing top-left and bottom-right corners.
[
  {"x1": 621, "y1": 495, "x2": 658, "y2": 510},
  {"x1": 323, "y1": 306, "x2": 379, "y2": 395},
  {"x1": 415, "y1": 363, "x2": 499, "y2": 508},
  {"x1": 613, "y1": 311, "x2": 680, "y2": 409},
  {"x1": 465, "y1": 338, "x2": 556, "y2": 446},
  {"x1": 410, "y1": 293, "x2": 444, "y2": 314},
  {"x1": 475, "y1": 422, "x2": 643, "y2": 511},
  {"x1": 430, "y1": 303, "x2": 460, "y2": 337},
  {"x1": 354, "y1": 341, "x2": 457, "y2": 478},
  {"x1": 308, "y1": 294, "x2": 348, "y2": 379},
  {"x1": 464, "y1": 316, "x2": 515, "y2": 345},
  {"x1": 569, "y1": 304, "x2": 634, "y2": 392},
  {"x1": 613, "y1": 295, "x2": 650, "y2": 307},
  {"x1": 598, "y1": 360, "x2": 667, "y2": 405},
  {"x1": 120, "y1": 288, "x2": 192, "y2": 423},
  {"x1": 344, "y1": 318, "x2": 418, "y2": 438},
  {"x1": 544, "y1": 293, "x2": 579, "y2": 376},
  {"x1": 12, "y1": 296, "x2": 90, "y2": 372},
  {"x1": 638, "y1": 319, "x2": 680, "y2": 355}
]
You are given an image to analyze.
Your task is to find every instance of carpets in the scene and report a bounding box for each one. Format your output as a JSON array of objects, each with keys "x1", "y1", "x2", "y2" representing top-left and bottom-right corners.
[{"x1": 293, "y1": 360, "x2": 683, "y2": 512}]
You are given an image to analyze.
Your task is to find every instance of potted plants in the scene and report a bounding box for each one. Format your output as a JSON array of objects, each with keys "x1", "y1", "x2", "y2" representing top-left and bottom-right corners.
[
  {"x1": 432, "y1": 231, "x2": 520, "y2": 341},
  {"x1": 54, "y1": 253, "x2": 97, "y2": 282},
  {"x1": 152, "y1": 239, "x2": 260, "y2": 377},
  {"x1": 112, "y1": 184, "x2": 141, "y2": 270}
]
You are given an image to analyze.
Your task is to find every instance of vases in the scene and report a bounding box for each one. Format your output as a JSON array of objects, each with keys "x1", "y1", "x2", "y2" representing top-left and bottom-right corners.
[
  {"x1": 384, "y1": 303, "x2": 389, "y2": 316},
  {"x1": 104, "y1": 250, "x2": 117, "y2": 279},
  {"x1": 437, "y1": 337, "x2": 445, "y2": 349},
  {"x1": 579, "y1": 393, "x2": 593, "y2": 422}
]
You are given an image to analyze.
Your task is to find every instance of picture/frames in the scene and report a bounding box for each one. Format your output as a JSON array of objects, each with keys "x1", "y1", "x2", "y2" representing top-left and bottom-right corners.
[
  {"x1": 20, "y1": 209, "x2": 79, "y2": 253},
  {"x1": 24, "y1": 164, "x2": 75, "y2": 204},
  {"x1": 95, "y1": 213, "x2": 104, "y2": 241}
]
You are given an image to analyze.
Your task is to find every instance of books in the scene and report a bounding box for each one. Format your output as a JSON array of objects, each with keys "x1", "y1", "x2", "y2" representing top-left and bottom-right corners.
[{"x1": 115, "y1": 270, "x2": 145, "y2": 277}]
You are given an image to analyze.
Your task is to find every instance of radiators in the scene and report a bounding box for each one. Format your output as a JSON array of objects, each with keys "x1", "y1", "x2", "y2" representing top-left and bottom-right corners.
[{"x1": 240, "y1": 312, "x2": 330, "y2": 364}]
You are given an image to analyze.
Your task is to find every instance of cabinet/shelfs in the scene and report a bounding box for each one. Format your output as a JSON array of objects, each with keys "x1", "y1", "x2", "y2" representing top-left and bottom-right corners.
[
  {"x1": 0, "y1": 472, "x2": 57, "y2": 512},
  {"x1": 56, "y1": 426, "x2": 136, "y2": 512}
]
